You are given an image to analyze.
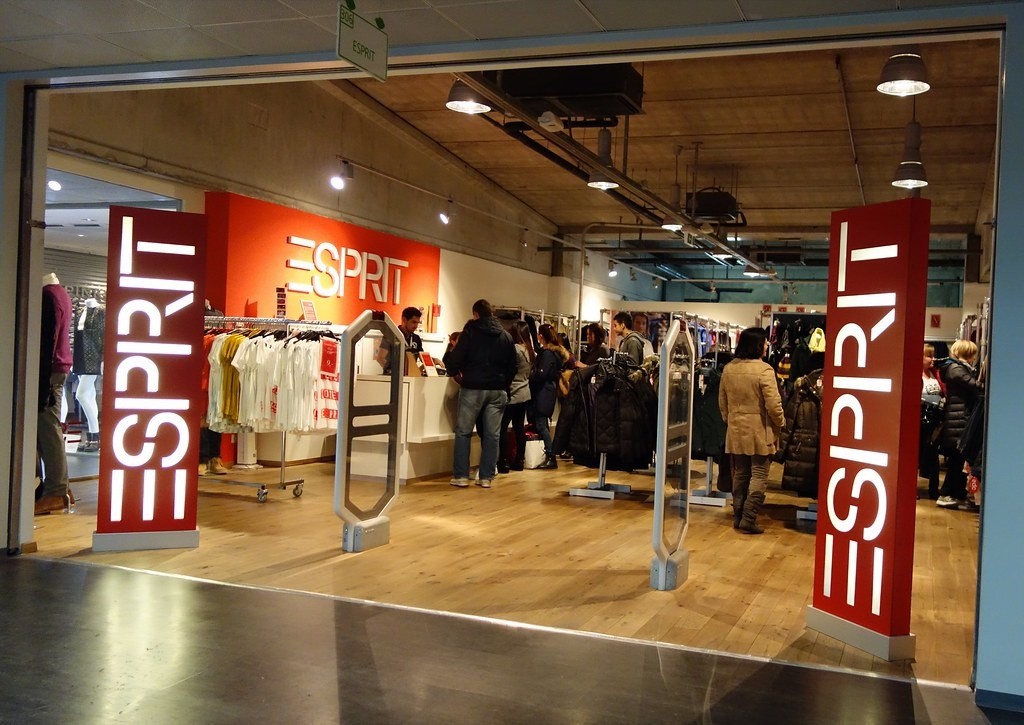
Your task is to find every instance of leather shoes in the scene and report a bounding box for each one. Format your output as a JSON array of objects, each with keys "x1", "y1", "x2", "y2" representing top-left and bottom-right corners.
[{"x1": 498, "y1": 459, "x2": 525, "y2": 473}]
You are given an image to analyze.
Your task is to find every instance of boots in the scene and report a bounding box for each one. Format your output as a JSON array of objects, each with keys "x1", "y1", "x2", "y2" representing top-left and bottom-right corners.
[
  {"x1": 76, "y1": 432, "x2": 101, "y2": 452},
  {"x1": 731, "y1": 489, "x2": 766, "y2": 534},
  {"x1": 537, "y1": 454, "x2": 558, "y2": 470},
  {"x1": 199, "y1": 457, "x2": 229, "y2": 475}
]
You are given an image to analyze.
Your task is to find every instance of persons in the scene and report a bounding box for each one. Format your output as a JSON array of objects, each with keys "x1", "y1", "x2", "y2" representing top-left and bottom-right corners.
[
  {"x1": 719, "y1": 327, "x2": 786, "y2": 533},
  {"x1": 916, "y1": 343, "x2": 946, "y2": 500},
  {"x1": 377, "y1": 306, "x2": 444, "y2": 374},
  {"x1": 574, "y1": 323, "x2": 610, "y2": 367},
  {"x1": 632, "y1": 313, "x2": 655, "y2": 359},
  {"x1": 444, "y1": 300, "x2": 517, "y2": 488},
  {"x1": 71, "y1": 298, "x2": 105, "y2": 453},
  {"x1": 496, "y1": 320, "x2": 532, "y2": 474},
  {"x1": 32, "y1": 273, "x2": 75, "y2": 515},
  {"x1": 936, "y1": 340, "x2": 986, "y2": 507},
  {"x1": 529, "y1": 325, "x2": 564, "y2": 469},
  {"x1": 446, "y1": 332, "x2": 461, "y2": 353},
  {"x1": 557, "y1": 332, "x2": 576, "y2": 459},
  {"x1": 613, "y1": 312, "x2": 644, "y2": 367}
]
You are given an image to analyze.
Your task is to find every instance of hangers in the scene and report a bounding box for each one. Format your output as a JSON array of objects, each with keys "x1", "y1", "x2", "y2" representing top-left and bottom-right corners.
[
  {"x1": 691, "y1": 368, "x2": 722, "y2": 381},
  {"x1": 502, "y1": 315, "x2": 571, "y2": 335},
  {"x1": 203, "y1": 315, "x2": 342, "y2": 351},
  {"x1": 594, "y1": 357, "x2": 640, "y2": 379},
  {"x1": 651, "y1": 312, "x2": 733, "y2": 336}
]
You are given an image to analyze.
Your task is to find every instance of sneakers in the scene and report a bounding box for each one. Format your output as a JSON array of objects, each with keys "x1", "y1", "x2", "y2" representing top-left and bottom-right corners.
[
  {"x1": 450, "y1": 478, "x2": 469, "y2": 487},
  {"x1": 475, "y1": 478, "x2": 491, "y2": 487},
  {"x1": 935, "y1": 495, "x2": 958, "y2": 508}
]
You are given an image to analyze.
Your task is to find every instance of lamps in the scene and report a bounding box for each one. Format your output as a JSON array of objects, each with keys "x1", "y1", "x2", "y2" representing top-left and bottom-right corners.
[
  {"x1": 651, "y1": 276, "x2": 659, "y2": 288},
  {"x1": 877, "y1": 40, "x2": 931, "y2": 189},
  {"x1": 439, "y1": 196, "x2": 453, "y2": 224},
  {"x1": 586, "y1": 128, "x2": 619, "y2": 190},
  {"x1": 629, "y1": 266, "x2": 637, "y2": 281},
  {"x1": 660, "y1": 146, "x2": 685, "y2": 231},
  {"x1": 445, "y1": 77, "x2": 491, "y2": 115},
  {"x1": 608, "y1": 259, "x2": 617, "y2": 277},
  {"x1": 742, "y1": 246, "x2": 758, "y2": 278},
  {"x1": 331, "y1": 158, "x2": 350, "y2": 190}
]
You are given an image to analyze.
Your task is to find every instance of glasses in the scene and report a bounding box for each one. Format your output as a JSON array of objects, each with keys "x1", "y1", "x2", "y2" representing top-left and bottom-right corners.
[{"x1": 613, "y1": 322, "x2": 620, "y2": 327}]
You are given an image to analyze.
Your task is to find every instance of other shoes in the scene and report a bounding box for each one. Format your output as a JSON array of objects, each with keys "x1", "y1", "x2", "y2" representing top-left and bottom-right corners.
[{"x1": 34, "y1": 490, "x2": 75, "y2": 515}]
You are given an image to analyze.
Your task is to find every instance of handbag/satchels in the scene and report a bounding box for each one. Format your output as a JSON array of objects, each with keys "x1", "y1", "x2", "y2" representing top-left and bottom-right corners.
[{"x1": 507, "y1": 424, "x2": 546, "y2": 469}]
